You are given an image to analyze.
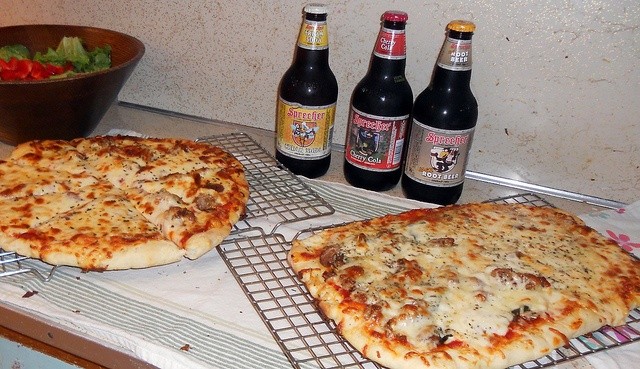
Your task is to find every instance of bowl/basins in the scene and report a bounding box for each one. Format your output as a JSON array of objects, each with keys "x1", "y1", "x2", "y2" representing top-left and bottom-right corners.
[{"x1": 0, "y1": 23, "x2": 145, "y2": 145}]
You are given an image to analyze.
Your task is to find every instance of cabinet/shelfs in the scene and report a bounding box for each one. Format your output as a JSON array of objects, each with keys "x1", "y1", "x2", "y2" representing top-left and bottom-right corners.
[{"x1": 0, "y1": 101, "x2": 640, "y2": 369}]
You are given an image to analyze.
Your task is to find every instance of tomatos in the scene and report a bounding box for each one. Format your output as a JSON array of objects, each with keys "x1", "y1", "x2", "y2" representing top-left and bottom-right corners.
[{"x1": 0, "y1": 58, "x2": 73, "y2": 81}]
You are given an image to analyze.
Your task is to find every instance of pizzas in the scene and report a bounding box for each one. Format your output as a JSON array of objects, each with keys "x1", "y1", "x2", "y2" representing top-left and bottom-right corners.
[
  {"x1": 287, "y1": 202, "x2": 640, "y2": 369},
  {"x1": 0, "y1": 135, "x2": 249, "y2": 270}
]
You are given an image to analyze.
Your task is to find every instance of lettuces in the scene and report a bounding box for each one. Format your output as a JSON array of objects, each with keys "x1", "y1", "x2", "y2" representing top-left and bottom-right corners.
[{"x1": 1, "y1": 37, "x2": 112, "y2": 79}]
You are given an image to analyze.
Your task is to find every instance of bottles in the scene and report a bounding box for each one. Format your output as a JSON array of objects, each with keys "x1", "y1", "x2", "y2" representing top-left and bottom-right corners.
[
  {"x1": 343, "y1": 10, "x2": 413, "y2": 191},
  {"x1": 275, "y1": 3, "x2": 338, "y2": 178},
  {"x1": 401, "y1": 20, "x2": 478, "y2": 204}
]
